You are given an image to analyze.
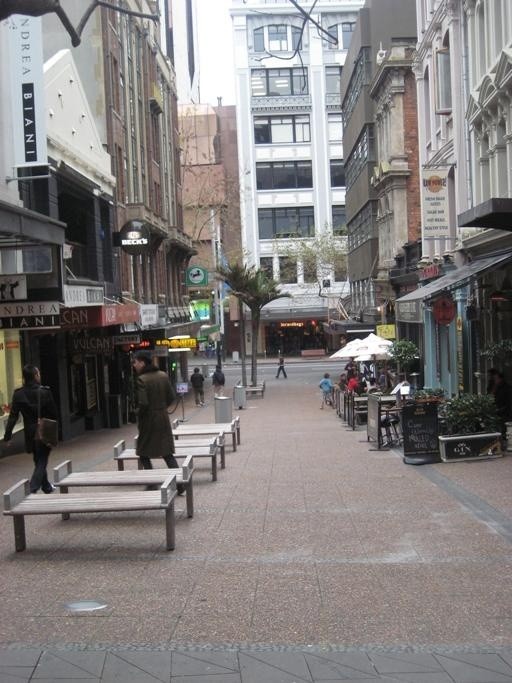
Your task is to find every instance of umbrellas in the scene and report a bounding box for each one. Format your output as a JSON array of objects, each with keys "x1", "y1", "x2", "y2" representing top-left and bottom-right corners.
[{"x1": 329, "y1": 332, "x2": 397, "y2": 364}]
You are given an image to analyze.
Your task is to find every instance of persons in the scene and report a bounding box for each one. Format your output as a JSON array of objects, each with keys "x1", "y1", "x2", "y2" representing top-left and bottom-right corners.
[
  {"x1": 191, "y1": 365, "x2": 206, "y2": 406},
  {"x1": 211, "y1": 362, "x2": 225, "y2": 402},
  {"x1": 3, "y1": 363, "x2": 64, "y2": 493},
  {"x1": 487, "y1": 368, "x2": 512, "y2": 452},
  {"x1": 315, "y1": 356, "x2": 397, "y2": 408},
  {"x1": 132, "y1": 348, "x2": 187, "y2": 497},
  {"x1": 274, "y1": 352, "x2": 288, "y2": 378}
]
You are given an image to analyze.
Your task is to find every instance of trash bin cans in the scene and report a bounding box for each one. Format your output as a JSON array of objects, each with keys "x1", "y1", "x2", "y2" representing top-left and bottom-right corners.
[
  {"x1": 213, "y1": 395, "x2": 234, "y2": 424},
  {"x1": 233, "y1": 385, "x2": 246, "y2": 410}
]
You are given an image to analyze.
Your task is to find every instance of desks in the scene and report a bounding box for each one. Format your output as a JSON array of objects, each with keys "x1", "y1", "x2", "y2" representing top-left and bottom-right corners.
[{"x1": 326, "y1": 379, "x2": 413, "y2": 448}]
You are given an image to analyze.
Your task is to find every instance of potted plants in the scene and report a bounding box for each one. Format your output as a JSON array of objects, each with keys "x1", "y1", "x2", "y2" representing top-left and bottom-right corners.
[{"x1": 438, "y1": 391, "x2": 503, "y2": 462}]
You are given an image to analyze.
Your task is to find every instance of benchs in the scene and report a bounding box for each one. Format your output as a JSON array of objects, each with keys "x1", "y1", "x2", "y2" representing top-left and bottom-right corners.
[
  {"x1": 134, "y1": 432, "x2": 225, "y2": 469},
  {"x1": 113, "y1": 438, "x2": 220, "y2": 481},
  {"x1": 3, "y1": 474, "x2": 181, "y2": 552},
  {"x1": 51, "y1": 452, "x2": 197, "y2": 520},
  {"x1": 233, "y1": 379, "x2": 266, "y2": 397},
  {"x1": 173, "y1": 417, "x2": 241, "y2": 445},
  {"x1": 171, "y1": 423, "x2": 236, "y2": 452}
]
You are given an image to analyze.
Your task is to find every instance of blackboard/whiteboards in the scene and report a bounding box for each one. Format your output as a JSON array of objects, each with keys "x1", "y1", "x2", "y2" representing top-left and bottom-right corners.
[{"x1": 400, "y1": 404, "x2": 440, "y2": 465}]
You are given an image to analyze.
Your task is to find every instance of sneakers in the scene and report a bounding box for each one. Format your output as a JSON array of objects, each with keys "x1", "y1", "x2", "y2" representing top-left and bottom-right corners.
[
  {"x1": 43, "y1": 485, "x2": 55, "y2": 493},
  {"x1": 144, "y1": 485, "x2": 157, "y2": 490},
  {"x1": 176, "y1": 484, "x2": 185, "y2": 494}
]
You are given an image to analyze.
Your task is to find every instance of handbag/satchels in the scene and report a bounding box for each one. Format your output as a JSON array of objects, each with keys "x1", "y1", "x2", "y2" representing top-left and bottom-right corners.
[{"x1": 34, "y1": 418, "x2": 58, "y2": 448}]
